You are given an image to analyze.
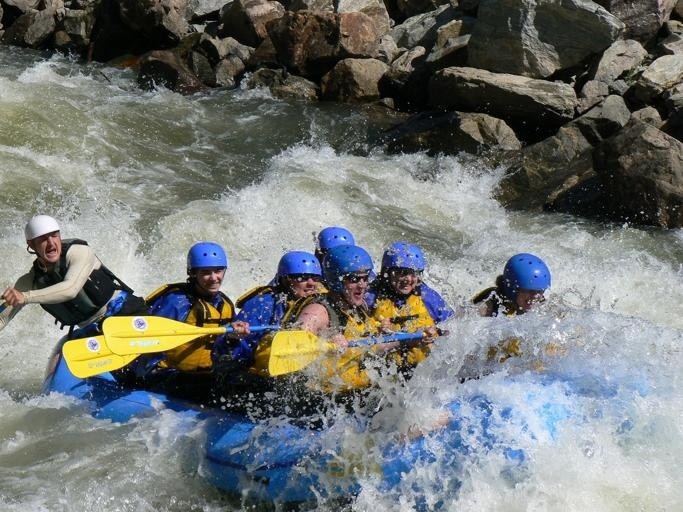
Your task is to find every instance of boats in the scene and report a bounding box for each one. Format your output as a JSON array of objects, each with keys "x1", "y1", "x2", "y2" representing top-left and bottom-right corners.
[{"x1": 43, "y1": 313, "x2": 668, "y2": 512}]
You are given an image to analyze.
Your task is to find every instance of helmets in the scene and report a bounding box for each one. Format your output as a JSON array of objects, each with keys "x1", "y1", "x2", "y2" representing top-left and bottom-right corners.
[
  {"x1": 23, "y1": 215, "x2": 60, "y2": 242},
  {"x1": 321, "y1": 244, "x2": 373, "y2": 293},
  {"x1": 500, "y1": 252, "x2": 551, "y2": 297},
  {"x1": 315, "y1": 227, "x2": 356, "y2": 258},
  {"x1": 276, "y1": 250, "x2": 322, "y2": 294},
  {"x1": 186, "y1": 241, "x2": 227, "y2": 277},
  {"x1": 380, "y1": 242, "x2": 425, "y2": 282}
]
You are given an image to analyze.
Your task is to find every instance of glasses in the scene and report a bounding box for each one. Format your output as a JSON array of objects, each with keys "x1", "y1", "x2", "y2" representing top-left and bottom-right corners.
[
  {"x1": 340, "y1": 272, "x2": 370, "y2": 283},
  {"x1": 287, "y1": 273, "x2": 321, "y2": 284}
]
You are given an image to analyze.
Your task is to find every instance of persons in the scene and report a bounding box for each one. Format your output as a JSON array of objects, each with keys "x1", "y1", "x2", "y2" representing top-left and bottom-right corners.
[
  {"x1": 364, "y1": 242, "x2": 454, "y2": 383},
  {"x1": 454, "y1": 251, "x2": 583, "y2": 384},
  {"x1": 144, "y1": 242, "x2": 235, "y2": 402},
  {"x1": 294, "y1": 244, "x2": 434, "y2": 430},
  {"x1": 0, "y1": 216, "x2": 145, "y2": 384},
  {"x1": 210, "y1": 251, "x2": 324, "y2": 416},
  {"x1": 315, "y1": 226, "x2": 376, "y2": 309}
]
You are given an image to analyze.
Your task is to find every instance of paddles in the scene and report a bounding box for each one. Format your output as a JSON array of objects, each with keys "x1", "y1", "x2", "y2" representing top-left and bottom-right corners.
[
  {"x1": 61, "y1": 334, "x2": 146, "y2": 379},
  {"x1": 268, "y1": 329, "x2": 436, "y2": 377},
  {"x1": 102, "y1": 314, "x2": 285, "y2": 356}
]
